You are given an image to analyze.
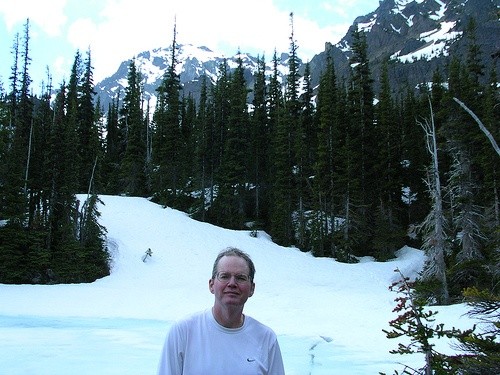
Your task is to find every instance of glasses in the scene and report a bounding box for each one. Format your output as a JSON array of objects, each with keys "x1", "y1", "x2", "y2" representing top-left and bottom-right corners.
[{"x1": 215, "y1": 271, "x2": 252, "y2": 284}]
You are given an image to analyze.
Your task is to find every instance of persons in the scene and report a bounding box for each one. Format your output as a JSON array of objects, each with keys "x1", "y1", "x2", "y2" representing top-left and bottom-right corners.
[{"x1": 157, "y1": 248, "x2": 286, "y2": 375}]
